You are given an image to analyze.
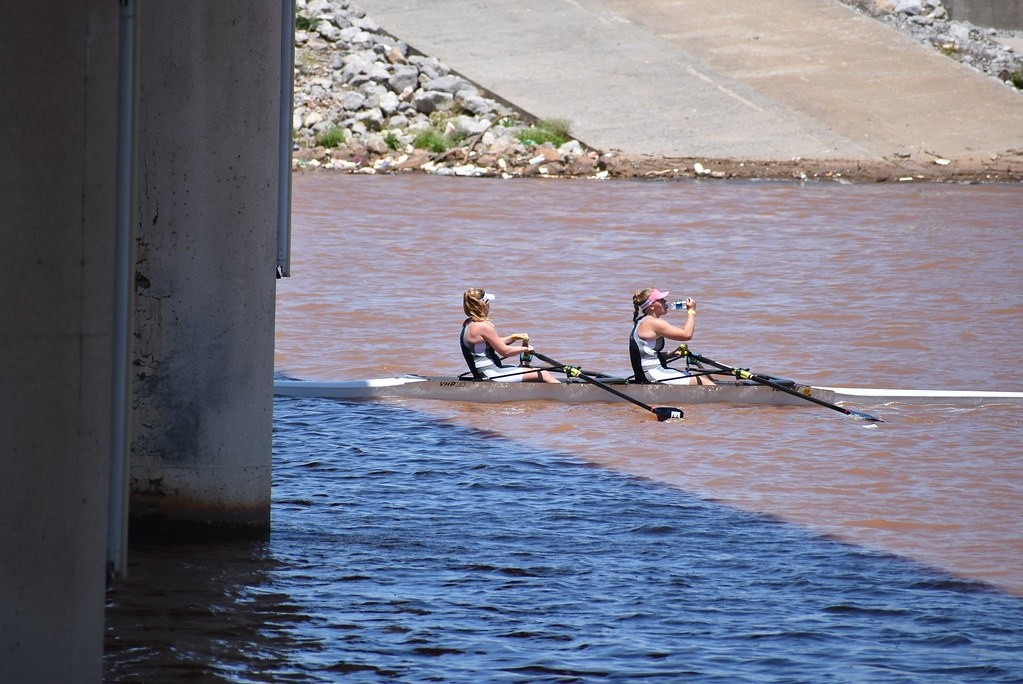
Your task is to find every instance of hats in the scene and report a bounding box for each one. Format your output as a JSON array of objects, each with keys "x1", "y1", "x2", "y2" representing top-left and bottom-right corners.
[
  {"x1": 479, "y1": 291, "x2": 494, "y2": 303},
  {"x1": 640, "y1": 289, "x2": 669, "y2": 309}
]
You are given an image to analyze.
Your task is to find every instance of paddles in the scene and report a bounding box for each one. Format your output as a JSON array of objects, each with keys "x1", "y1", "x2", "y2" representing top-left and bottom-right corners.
[
  {"x1": 680, "y1": 348, "x2": 895, "y2": 429},
  {"x1": 521, "y1": 341, "x2": 686, "y2": 422}
]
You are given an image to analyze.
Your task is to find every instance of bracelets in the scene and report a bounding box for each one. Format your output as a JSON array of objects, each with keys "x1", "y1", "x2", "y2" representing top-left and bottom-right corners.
[
  {"x1": 512, "y1": 334, "x2": 518, "y2": 341},
  {"x1": 688, "y1": 310, "x2": 696, "y2": 315}
]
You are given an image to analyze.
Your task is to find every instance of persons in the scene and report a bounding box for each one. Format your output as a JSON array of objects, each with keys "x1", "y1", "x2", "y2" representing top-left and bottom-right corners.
[
  {"x1": 629, "y1": 287, "x2": 717, "y2": 386},
  {"x1": 460, "y1": 289, "x2": 561, "y2": 384}
]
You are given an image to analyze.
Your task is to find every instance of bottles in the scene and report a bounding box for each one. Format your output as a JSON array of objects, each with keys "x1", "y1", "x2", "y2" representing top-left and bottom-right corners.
[{"x1": 666, "y1": 300, "x2": 688, "y2": 310}]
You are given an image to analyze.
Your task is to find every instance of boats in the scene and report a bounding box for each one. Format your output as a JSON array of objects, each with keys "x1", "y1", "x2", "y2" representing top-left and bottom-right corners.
[{"x1": 272, "y1": 368, "x2": 1023, "y2": 406}]
